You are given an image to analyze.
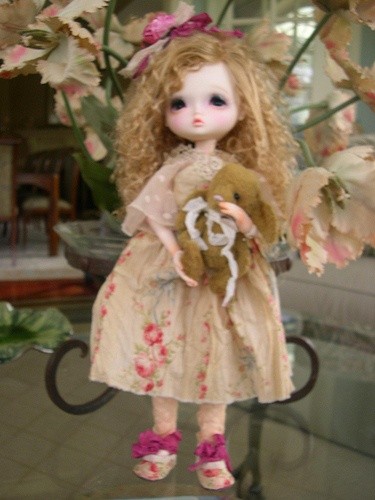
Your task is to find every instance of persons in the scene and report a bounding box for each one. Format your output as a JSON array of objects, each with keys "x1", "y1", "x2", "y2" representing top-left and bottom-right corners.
[{"x1": 89, "y1": 12, "x2": 298, "y2": 491}]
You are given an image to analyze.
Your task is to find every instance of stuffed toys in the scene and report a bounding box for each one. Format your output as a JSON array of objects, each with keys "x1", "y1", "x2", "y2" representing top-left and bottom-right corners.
[{"x1": 172, "y1": 164, "x2": 275, "y2": 292}]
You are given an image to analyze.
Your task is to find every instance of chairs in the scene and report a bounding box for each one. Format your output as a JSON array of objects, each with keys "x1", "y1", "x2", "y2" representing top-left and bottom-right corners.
[{"x1": 15, "y1": 162, "x2": 80, "y2": 257}]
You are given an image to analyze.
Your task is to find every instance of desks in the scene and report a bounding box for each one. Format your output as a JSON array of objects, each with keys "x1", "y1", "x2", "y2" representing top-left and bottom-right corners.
[{"x1": 0, "y1": 135, "x2": 25, "y2": 254}]
